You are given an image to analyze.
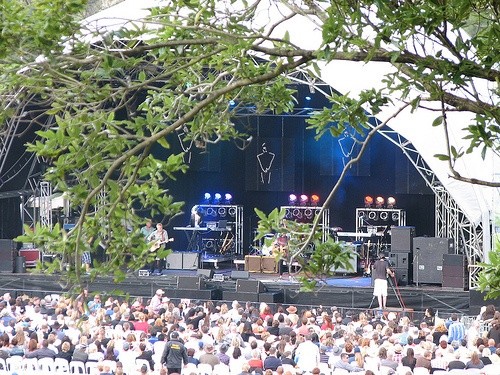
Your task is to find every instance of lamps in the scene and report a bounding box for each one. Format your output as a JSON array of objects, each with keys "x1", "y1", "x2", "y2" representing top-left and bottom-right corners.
[
  {"x1": 285, "y1": 195, "x2": 321, "y2": 219},
  {"x1": 198, "y1": 193, "x2": 236, "y2": 217},
  {"x1": 206, "y1": 241, "x2": 218, "y2": 252},
  {"x1": 358, "y1": 196, "x2": 399, "y2": 222}
]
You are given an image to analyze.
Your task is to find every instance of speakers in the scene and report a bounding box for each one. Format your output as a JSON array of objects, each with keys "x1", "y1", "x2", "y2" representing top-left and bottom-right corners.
[
  {"x1": 166, "y1": 252, "x2": 215, "y2": 290},
  {"x1": 324, "y1": 244, "x2": 361, "y2": 274},
  {"x1": 231, "y1": 255, "x2": 278, "y2": 293},
  {"x1": 389, "y1": 226, "x2": 469, "y2": 291},
  {"x1": 0, "y1": 239, "x2": 18, "y2": 272}
]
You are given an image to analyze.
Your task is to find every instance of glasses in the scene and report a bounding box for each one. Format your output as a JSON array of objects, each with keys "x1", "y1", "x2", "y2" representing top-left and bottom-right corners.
[{"x1": 346, "y1": 357, "x2": 349, "y2": 359}]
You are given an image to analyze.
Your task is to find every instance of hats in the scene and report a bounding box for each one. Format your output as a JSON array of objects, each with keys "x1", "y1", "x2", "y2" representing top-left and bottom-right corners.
[
  {"x1": 354, "y1": 348, "x2": 360, "y2": 353},
  {"x1": 123, "y1": 343, "x2": 129, "y2": 350},
  {"x1": 333, "y1": 346, "x2": 342, "y2": 355},
  {"x1": 162, "y1": 297, "x2": 170, "y2": 302},
  {"x1": 156, "y1": 289, "x2": 165, "y2": 295},
  {"x1": 286, "y1": 306, "x2": 297, "y2": 313},
  {"x1": 219, "y1": 343, "x2": 228, "y2": 353},
  {"x1": 172, "y1": 332, "x2": 179, "y2": 338},
  {"x1": 377, "y1": 252, "x2": 385, "y2": 258},
  {"x1": 204, "y1": 343, "x2": 214, "y2": 353},
  {"x1": 51, "y1": 300, "x2": 57, "y2": 306},
  {"x1": 104, "y1": 301, "x2": 112, "y2": 307},
  {"x1": 321, "y1": 345, "x2": 327, "y2": 351}
]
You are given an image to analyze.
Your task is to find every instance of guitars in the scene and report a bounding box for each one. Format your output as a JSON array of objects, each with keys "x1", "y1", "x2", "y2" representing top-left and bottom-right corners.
[{"x1": 146, "y1": 237, "x2": 175, "y2": 252}]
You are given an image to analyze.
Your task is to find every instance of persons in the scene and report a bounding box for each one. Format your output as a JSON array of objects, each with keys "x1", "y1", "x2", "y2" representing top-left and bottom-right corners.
[
  {"x1": 373, "y1": 253, "x2": 395, "y2": 309},
  {"x1": 149, "y1": 223, "x2": 168, "y2": 274},
  {"x1": 140, "y1": 219, "x2": 156, "y2": 239},
  {"x1": 277, "y1": 232, "x2": 288, "y2": 276},
  {"x1": 81, "y1": 236, "x2": 93, "y2": 274},
  {"x1": 0, "y1": 288, "x2": 500, "y2": 375}
]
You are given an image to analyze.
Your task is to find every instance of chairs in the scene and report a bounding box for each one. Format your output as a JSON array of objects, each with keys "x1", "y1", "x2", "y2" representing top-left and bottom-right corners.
[{"x1": 0, "y1": 357, "x2": 117, "y2": 375}]
